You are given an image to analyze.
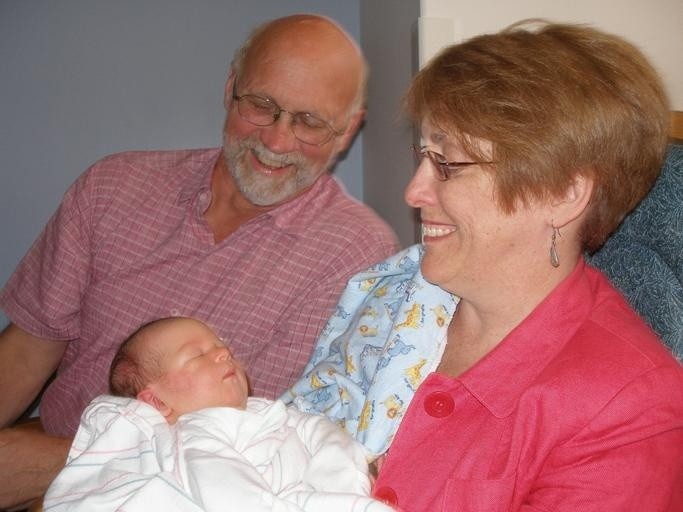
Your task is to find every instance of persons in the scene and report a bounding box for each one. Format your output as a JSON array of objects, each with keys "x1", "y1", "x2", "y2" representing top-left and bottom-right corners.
[
  {"x1": 0, "y1": 15, "x2": 402, "y2": 511},
  {"x1": 107, "y1": 317, "x2": 360, "y2": 511},
  {"x1": 359, "y1": 20, "x2": 683, "y2": 512}
]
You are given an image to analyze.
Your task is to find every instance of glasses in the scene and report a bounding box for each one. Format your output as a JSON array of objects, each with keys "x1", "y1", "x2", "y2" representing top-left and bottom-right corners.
[
  {"x1": 232, "y1": 77, "x2": 335, "y2": 147},
  {"x1": 411, "y1": 144, "x2": 494, "y2": 182}
]
